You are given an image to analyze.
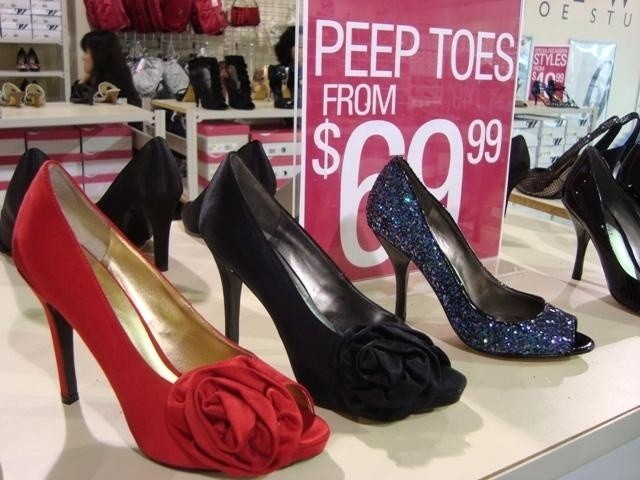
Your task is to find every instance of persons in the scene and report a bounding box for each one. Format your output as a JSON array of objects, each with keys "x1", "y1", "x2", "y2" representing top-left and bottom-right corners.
[
  {"x1": 78, "y1": 29, "x2": 144, "y2": 133},
  {"x1": 271, "y1": 25, "x2": 302, "y2": 108}
]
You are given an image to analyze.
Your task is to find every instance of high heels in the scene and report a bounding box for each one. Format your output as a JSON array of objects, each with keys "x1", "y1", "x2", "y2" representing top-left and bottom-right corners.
[
  {"x1": 198, "y1": 151, "x2": 466, "y2": 422},
  {"x1": 1, "y1": 148, "x2": 53, "y2": 253},
  {"x1": 98, "y1": 137, "x2": 180, "y2": 273},
  {"x1": 191, "y1": 57, "x2": 229, "y2": 109},
  {"x1": 24, "y1": 83, "x2": 46, "y2": 107},
  {"x1": 94, "y1": 82, "x2": 120, "y2": 104},
  {"x1": 532, "y1": 79, "x2": 579, "y2": 107},
  {"x1": 182, "y1": 141, "x2": 277, "y2": 238},
  {"x1": 506, "y1": 112, "x2": 640, "y2": 315},
  {"x1": 11, "y1": 158, "x2": 330, "y2": 479},
  {"x1": 367, "y1": 154, "x2": 594, "y2": 357},
  {"x1": 0, "y1": 82, "x2": 25, "y2": 107}
]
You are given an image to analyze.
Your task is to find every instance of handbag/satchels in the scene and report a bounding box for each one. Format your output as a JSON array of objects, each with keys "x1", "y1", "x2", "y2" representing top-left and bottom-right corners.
[
  {"x1": 230, "y1": 2, "x2": 261, "y2": 26},
  {"x1": 83, "y1": 0, "x2": 228, "y2": 35}
]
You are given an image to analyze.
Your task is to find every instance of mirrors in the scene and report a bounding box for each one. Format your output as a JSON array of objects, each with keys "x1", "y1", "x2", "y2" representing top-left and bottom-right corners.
[{"x1": 511, "y1": 33, "x2": 536, "y2": 104}]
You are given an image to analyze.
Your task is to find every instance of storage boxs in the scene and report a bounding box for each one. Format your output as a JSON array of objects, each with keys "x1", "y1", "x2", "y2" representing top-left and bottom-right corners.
[
  {"x1": 0, "y1": 97, "x2": 602, "y2": 205},
  {"x1": 0, "y1": 0, "x2": 64, "y2": 42}
]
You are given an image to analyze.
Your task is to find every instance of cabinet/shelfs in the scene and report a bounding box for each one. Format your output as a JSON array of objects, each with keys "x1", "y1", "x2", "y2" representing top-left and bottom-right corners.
[{"x1": 0, "y1": 0, "x2": 73, "y2": 104}]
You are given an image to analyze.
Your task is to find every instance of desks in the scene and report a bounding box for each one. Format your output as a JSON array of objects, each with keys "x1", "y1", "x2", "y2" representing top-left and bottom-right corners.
[
  {"x1": 509, "y1": 188, "x2": 573, "y2": 224},
  {"x1": 0, "y1": 199, "x2": 638, "y2": 480}
]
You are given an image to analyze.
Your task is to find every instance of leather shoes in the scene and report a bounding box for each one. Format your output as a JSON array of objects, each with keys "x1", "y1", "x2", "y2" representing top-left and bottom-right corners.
[
  {"x1": 29, "y1": 47, "x2": 41, "y2": 71},
  {"x1": 268, "y1": 64, "x2": 293, "y2": 108},
  {"x1": 224, "y1": 55, "x2": 255, "y2": 110},
  {"x1": 17, "y1": 47, "x2": 28, "y2": 71}
]
